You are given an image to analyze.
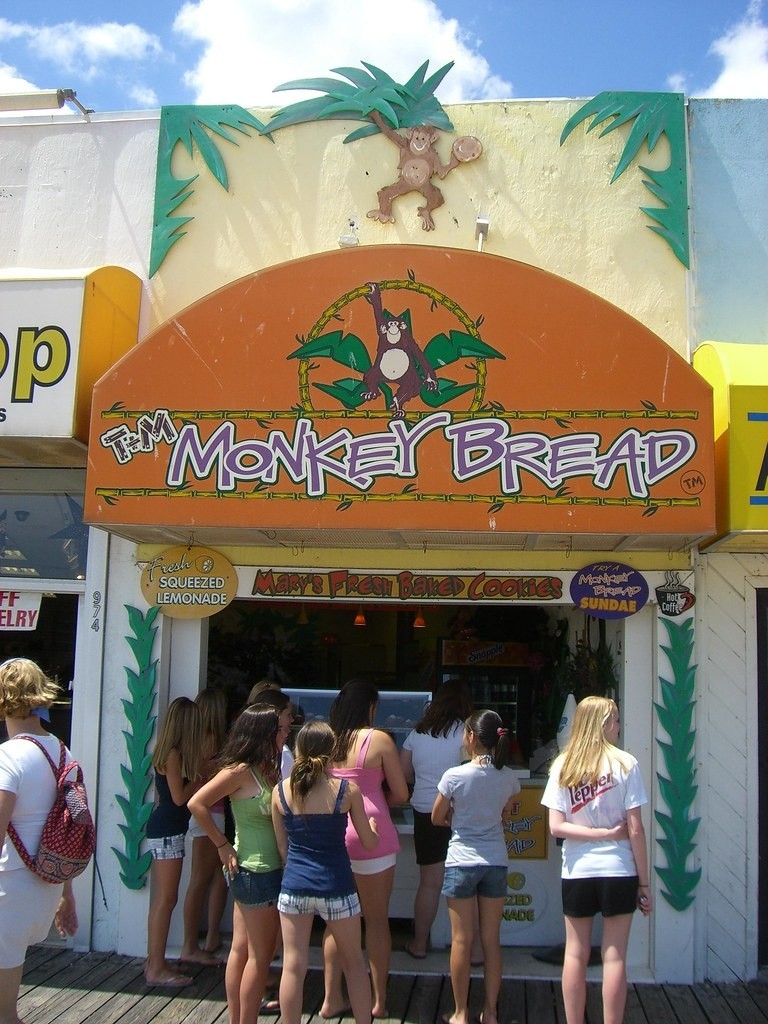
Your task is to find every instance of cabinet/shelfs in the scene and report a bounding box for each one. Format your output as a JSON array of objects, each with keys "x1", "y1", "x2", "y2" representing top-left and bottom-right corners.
[{"x1": 278, "y1": 689, "x2": 434, "y2": 834}]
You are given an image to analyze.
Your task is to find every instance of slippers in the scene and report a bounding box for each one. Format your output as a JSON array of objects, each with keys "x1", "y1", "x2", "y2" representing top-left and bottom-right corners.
[
  {"x1": 146, "y1": 972, "x2": 191, "y2": 987},
  {"x1": 408, "y1": 942, "x2": 426, "y2": 958},
  {"x1": 470, "y1": 960, "x2": 488, "y2": 965}
]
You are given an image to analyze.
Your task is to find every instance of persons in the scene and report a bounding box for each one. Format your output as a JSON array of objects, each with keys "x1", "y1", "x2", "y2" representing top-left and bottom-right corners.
[
  {"x1": 185, "y1": 702, "x2": 286, "y2": 1024},
  {"x1": 1, "y1": 657, "x2": 79, "y2": 1023},
  {"x1": 540, "y1": 696, "x2": 653, "y2": 1024},
  {"x1": 272, "y1": 721, "x2": 380, "y2": 1023},
  {"x1": 432, "y1": 709, "x2": 521, "y2": 1023},
  {"x1": 399, "y1": 678, "x2": 485, "y2": 968},
  {"x1": 143, "y1": 678, "x2": 295, "y2": 984},
  {"x1": 320, "y1": 675, "x2": 408, "y2": 1018}
]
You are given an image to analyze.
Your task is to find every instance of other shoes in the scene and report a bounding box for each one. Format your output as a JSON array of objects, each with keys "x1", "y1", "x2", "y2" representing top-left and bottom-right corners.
[
  {"x1": 211, "y1": 943, "x2": 228, "y2": 956},
  {"x1": 177, "y1": 949, "x2": 224, "y2": 965},
  {"x1": 259, "y1": 1000, "x2": 280, "y2": 1016}
]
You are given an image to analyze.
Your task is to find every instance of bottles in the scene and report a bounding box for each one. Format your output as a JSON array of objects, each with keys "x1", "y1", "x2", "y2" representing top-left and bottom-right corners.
[{"x1": 444, "y1": 670, "x2": 516, "y2": 735}]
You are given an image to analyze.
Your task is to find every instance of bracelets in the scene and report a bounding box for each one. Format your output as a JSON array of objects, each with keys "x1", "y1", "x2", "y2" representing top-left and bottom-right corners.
[
  {"x1": 217, "y1": 841, "x2": 229, "y2": 848},
  {"x1": 639, "y1": 884, "x2": 648, "y2": 887}
]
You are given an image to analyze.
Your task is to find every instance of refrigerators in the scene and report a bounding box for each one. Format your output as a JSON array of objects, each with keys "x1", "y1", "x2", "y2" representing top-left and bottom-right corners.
[{"x1": 438, "y1": 636, "x2": 533, "y2": 766}]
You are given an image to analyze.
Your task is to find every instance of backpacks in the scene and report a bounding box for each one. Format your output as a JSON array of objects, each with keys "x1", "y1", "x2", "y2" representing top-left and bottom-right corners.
[{"x1": 5, "y1": 736, "x2": 95, "y2": 884}]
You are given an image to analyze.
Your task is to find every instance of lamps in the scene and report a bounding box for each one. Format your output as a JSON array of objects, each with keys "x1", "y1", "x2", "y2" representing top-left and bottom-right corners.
[
  {"x1": 413, "y1": 604, "x2": 427, "y2": 628},
  {"x1": 296, "y1": 603, "x2": 310, "y2": 625},
  {"x1": 353, "y1": 603, "x2": 365, "y2": 626}
]
